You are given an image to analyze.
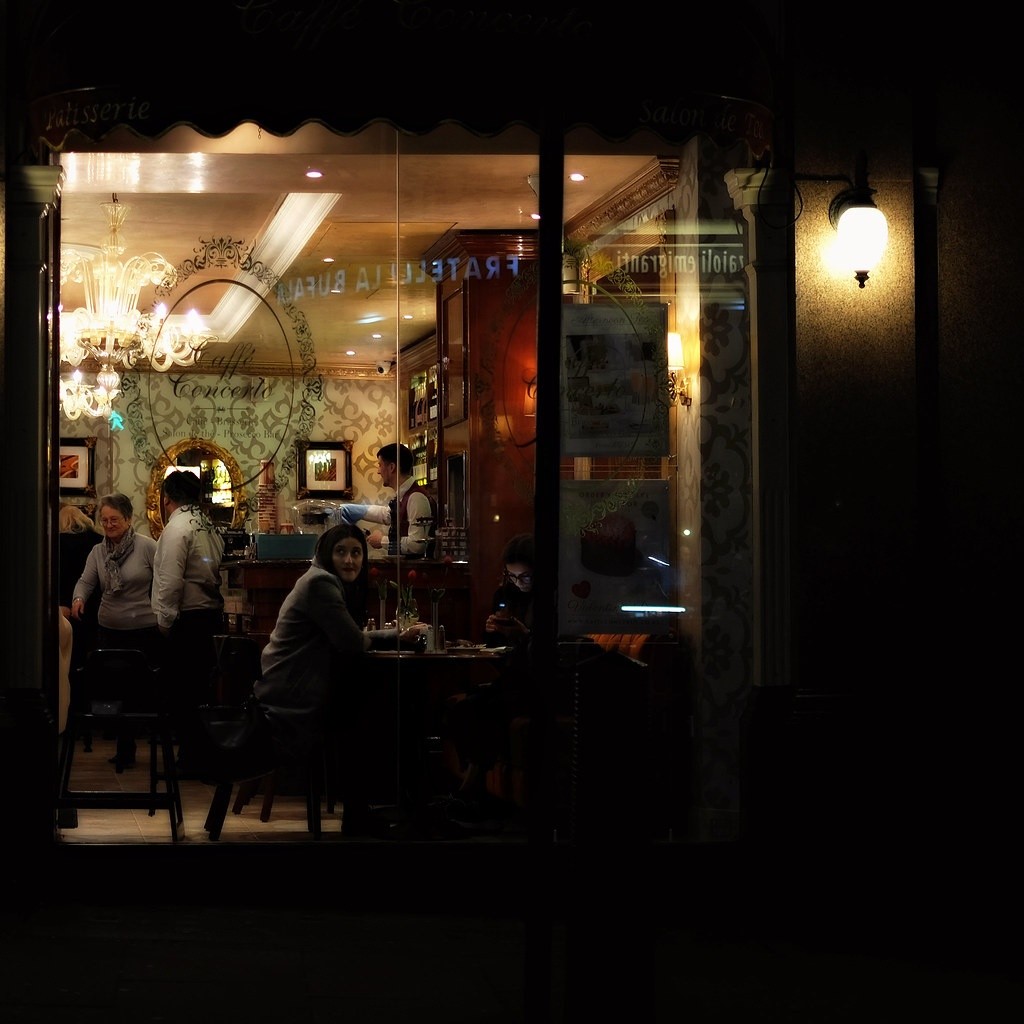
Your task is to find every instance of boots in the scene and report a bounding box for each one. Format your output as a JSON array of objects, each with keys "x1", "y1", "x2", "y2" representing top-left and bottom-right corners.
[{"x1": 342, "y1": 790, "x2": 392, "y2": 837}]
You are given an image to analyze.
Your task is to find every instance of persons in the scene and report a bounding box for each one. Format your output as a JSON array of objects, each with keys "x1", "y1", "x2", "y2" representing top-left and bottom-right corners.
[
  {"x1": 60, "y1": 443, "x2": 537, "y2": 835},
  {"x1": 72, "y1": 494, "x2": 159, "y2": 763}
]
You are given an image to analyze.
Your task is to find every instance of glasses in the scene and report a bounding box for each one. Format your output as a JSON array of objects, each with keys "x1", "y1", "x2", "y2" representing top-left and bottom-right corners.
[
  {"x1": 98, "y1": 514, "x2": 125, "y2": 526},
  {"x1": 503, "y1": 569, "x2": 534, "y2": 584}
]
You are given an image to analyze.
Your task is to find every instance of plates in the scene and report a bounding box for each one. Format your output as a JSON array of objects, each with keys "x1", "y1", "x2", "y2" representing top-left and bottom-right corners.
[{"x1": 447, "y1": 645, "x2": 483, "y2": 654}]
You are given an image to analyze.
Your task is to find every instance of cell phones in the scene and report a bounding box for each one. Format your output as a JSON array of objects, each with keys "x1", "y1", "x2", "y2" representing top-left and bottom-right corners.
[{"x1": 493, "y1": 617, "x2": 515, "y2": 627}]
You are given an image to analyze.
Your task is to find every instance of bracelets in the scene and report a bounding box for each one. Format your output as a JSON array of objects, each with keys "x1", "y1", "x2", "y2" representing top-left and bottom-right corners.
[{"x1": 73, "y1": 598, "x2": 83, "y2": 604}]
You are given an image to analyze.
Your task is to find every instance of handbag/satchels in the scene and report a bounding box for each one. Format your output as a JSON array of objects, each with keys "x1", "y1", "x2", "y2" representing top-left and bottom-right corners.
[{"x1": 193, "y1": 634, "x2": 275, "y2": 786}]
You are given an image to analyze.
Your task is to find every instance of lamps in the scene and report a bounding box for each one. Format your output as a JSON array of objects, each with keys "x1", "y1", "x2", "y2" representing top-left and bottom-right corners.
[
  {"x1": 827, "y1": 186, "x2": 892, "y2": 289},
  {"x1": 58, "y1": 193, "x2": 213, "y2": 422}
]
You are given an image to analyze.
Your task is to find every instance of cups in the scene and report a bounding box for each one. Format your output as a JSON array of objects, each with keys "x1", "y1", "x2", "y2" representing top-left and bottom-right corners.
[
  {"x1": 279, "y1": 523, "x2": 295, "y2": 535},
  {"x1": 257, "y1": 460, "x2": 277, "y2": 535}
]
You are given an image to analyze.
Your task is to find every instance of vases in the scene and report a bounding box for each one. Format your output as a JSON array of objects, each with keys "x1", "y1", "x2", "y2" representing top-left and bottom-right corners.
[
  {"x1": 379, "y1": 599, "x2": 387, "y2": 630},
  {"x1": 430, "y1": 601, "x2": 439, "y2": 650},
  {"x1": 395, "y1": 604, "x2": 419, "y2": 630}
]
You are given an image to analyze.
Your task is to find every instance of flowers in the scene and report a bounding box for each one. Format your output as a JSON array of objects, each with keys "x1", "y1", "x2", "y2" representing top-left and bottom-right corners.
[
  {"x1": 420, "y1": 555, "x2": 454, "y2": 603},
  {"x1": 370, "y1": 567, "x2": 389, "y2": 599},
  {"x1": 388, "y1": 569, "x2": 418, "y2": 624}
]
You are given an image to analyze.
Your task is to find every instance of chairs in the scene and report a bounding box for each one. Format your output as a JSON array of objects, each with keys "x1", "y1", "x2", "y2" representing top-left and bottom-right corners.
[
  {"x1": 54, "y1": 645, "x2": 183, "y2": 836},
  {"x1": 198, "y1": 634, "x2": 337, "y2": 836}
]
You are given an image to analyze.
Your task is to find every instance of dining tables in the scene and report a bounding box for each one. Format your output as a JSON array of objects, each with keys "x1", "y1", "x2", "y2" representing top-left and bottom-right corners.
[{"x1": 352, "y1": 641, "x2": 514, "y2": 824}]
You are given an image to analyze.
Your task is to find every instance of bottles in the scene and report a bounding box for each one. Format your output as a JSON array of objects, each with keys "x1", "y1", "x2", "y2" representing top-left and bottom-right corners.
[
  {"x1": 437, "y1": 626, "x2": 447, "y2": 649},
  {"x1": 430, "y1": 438, "x2": 438, "y2": 482},
  {"x1": 411, "y1": 383, "x2": 427, "y2": 426},
  {"x1": 427, "y1": 626, "x2": 433, "y2": 651},
  {"x1": 428, "y1": 376, "x2": 438, "y2": 420},
  {"x1": 408, "y1": 430, "x2": 427, "y2": 485}
]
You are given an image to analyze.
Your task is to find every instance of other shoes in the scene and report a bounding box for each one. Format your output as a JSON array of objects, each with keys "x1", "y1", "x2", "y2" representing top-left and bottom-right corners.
[{"x1": 107, "y1": 755, "x2": 136, "y2": 768}]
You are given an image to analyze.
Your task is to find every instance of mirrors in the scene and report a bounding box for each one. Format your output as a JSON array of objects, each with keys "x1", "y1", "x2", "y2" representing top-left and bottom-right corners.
[{"x1": 145, "y1": 438, "x2": 246, "y2": 543}]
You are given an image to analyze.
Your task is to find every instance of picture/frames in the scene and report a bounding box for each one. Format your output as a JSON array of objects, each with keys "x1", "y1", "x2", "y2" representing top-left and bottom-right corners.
[
  {"x1": 59, "y1": 436, "x2": 98, "y2": 498},
  {"x1": 295, "y1": 438, "x2": 355, "y2": 500}
]
